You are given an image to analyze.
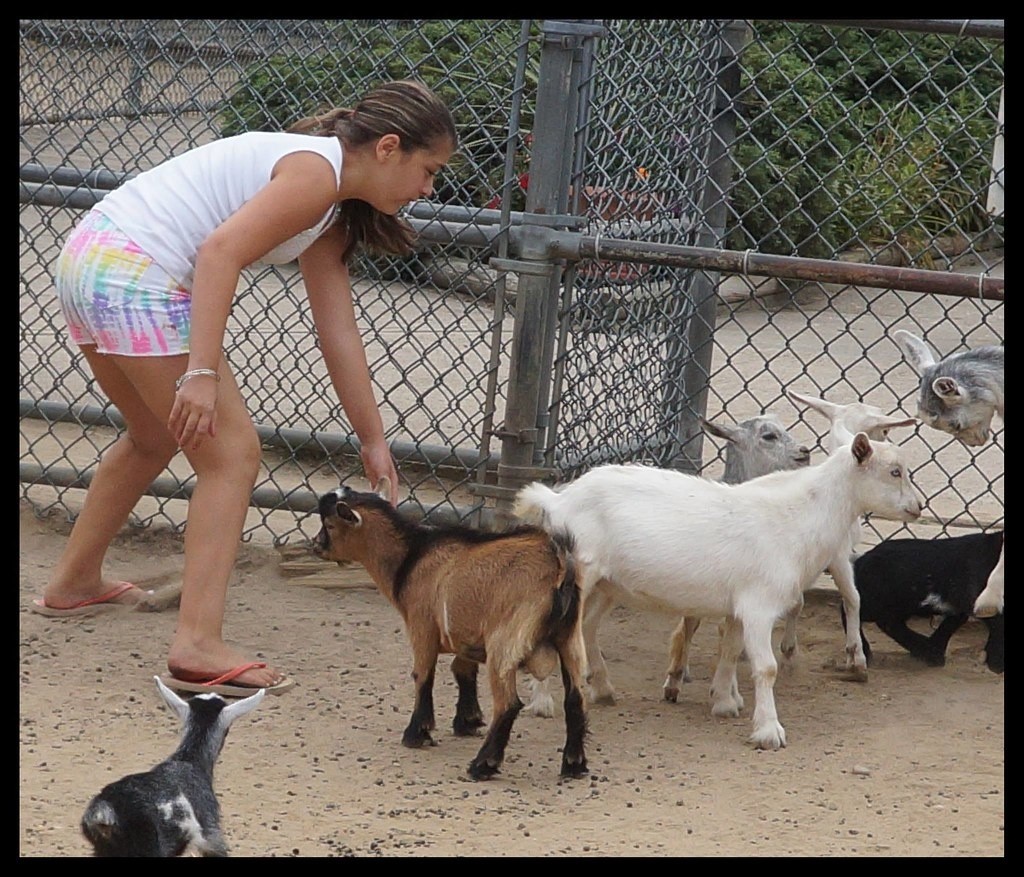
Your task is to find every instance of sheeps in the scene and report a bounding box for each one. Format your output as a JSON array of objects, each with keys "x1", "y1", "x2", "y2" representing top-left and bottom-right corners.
[
  {"x1": 840, "y1": 531, "x2": 1005, "y2": 677},
  {"x1": 894, "y1": 326, "x2": 1005, "y2": 619},
  {"x1": 80, "y1": 676, "x2": 266, "y2": 857},
  {"x1": 505, "y1": 390, "x2": 928, "y2": 751},
  {"x1": 310, "y1": 476, "x2": 591, "y2": 780}
]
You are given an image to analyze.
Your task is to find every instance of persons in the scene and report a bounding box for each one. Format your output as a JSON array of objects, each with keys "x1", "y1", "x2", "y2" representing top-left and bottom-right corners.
[{"x1": 31, "y1": 78, "x2": 460, "y2": 698}]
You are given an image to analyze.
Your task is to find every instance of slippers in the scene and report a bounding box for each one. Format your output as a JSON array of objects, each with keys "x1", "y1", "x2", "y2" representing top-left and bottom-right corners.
[
  {"x1": 32, "y1": 581, "x2": 135, "y2": 616},
  {"x1": 161, "y1": 661, "x2": 295, "y2": 696}
]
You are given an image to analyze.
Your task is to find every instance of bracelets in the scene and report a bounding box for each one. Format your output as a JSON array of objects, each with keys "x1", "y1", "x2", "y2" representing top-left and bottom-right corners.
[{"x1": 175, "y1": 367, "x2": 221, "y2": 386}]
